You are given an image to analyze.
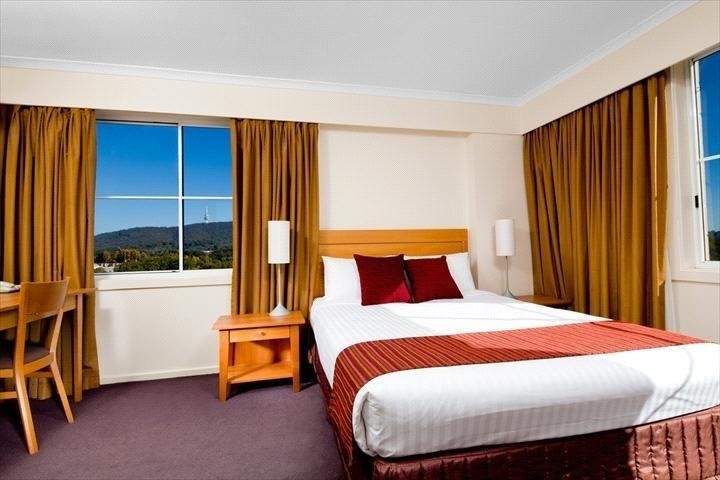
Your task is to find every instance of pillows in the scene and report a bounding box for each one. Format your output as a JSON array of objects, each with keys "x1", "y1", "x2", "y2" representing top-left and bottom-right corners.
[
  {"x1": 354, "y1": 252, "x2": 414, "y2": 304},
  {"x1": 404, "y1": 256, "x2": 465, "y2": 302},
  {"x1": 322, "y1": 253, "x2": 478, "y2": 298}
]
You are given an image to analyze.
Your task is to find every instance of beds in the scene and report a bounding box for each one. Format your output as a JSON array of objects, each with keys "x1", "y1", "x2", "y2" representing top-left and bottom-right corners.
[{"x1": 310, "y1": 230, "x2": 720, "y2": 480}]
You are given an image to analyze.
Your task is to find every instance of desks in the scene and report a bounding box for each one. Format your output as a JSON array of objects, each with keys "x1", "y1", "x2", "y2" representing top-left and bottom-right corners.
[{"x1": 0, "y1": 287, "x2": 96, "y2": 401}]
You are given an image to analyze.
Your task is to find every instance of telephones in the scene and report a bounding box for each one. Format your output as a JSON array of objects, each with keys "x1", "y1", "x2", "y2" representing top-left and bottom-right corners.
[{"x1": 0, "y1": 281, "x2": 20, "y2": 292}]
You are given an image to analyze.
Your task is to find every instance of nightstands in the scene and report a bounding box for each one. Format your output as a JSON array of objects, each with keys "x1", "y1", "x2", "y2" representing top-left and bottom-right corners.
[
  {"x1": 507, "y1": 294, "x2": 571, "y2": 305},
  {"x1": 214, "y1": 311, "x2": 304, "y2": 400}
]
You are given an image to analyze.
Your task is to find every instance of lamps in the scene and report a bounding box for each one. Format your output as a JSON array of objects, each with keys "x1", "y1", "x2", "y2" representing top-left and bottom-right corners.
[
  {"x1": 268, "y1": 220, "x2": 291, "y2": 316},
  {"x1": 494, "y1": 219, "x2": 515, "y2": 298}
]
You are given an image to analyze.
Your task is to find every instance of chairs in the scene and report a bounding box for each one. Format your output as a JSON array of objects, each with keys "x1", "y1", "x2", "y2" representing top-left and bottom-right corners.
[{"x1": 0, "y1": 278, "x2": 73, "y2": 453}]
